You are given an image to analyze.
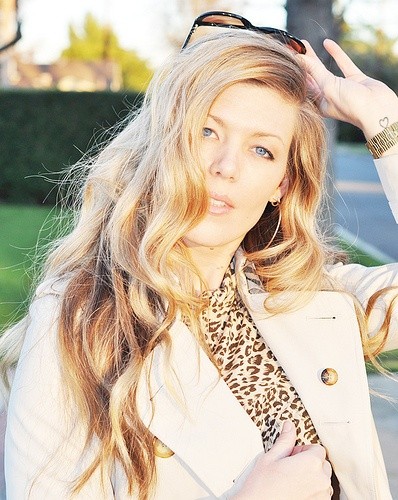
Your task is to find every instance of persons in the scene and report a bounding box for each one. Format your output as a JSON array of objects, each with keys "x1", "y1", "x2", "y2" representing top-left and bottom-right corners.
[{"x1": 0, "y1": 29, "x2": 398, "y2": 500}]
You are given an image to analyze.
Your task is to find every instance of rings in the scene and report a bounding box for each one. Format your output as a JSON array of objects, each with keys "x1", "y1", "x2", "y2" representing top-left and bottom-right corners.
[{"x1": 330, "y1": 486, "x2": 333, "y2": 496}]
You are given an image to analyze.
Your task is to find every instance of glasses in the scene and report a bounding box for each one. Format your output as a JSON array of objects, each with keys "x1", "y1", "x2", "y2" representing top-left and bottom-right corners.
[{"x1": 179, "y1": 11, "x2": 307, "y2": 55}]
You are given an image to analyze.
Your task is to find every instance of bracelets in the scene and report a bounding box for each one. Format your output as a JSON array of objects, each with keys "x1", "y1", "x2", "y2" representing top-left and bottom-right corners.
[{"x1": 366, "y1": 122, "x2": 398, "y2": 159}]
